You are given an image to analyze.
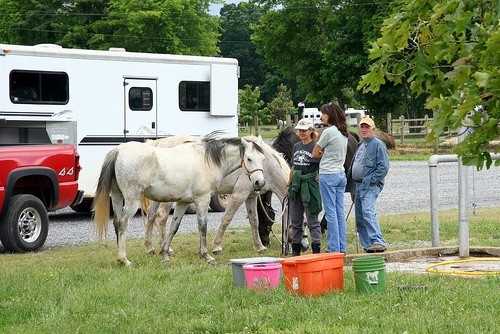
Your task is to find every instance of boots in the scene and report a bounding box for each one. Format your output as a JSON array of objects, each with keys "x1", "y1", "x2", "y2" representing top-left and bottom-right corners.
[
  {"x1": 311, "y1": 242, "x2": 321, "y2": 254},
  {"x1": 292, "y1": 242, "x2": 302, "y2": 256}
]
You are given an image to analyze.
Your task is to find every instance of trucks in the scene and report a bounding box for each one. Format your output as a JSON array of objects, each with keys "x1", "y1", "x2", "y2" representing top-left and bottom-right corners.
[
  {"x1": 302, "y1": 108, "x2": 365, "y2": 128},
  {"x1": 0, "y1": 42, "x2": 241, "y2": 212}
]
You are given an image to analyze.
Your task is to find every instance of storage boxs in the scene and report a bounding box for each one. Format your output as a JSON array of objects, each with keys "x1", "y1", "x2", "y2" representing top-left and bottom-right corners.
[
  {"x1": 279, "y1": 252, "x2": 346, "y2": 298},
  {"x1": 230, "y1": 256, "x2": 280, "y2": 288}
]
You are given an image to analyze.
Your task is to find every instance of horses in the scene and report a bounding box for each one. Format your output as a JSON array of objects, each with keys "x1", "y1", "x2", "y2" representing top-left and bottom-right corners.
[{"x1": 90, "y1": 127, "x2": 396, "y2": 266}]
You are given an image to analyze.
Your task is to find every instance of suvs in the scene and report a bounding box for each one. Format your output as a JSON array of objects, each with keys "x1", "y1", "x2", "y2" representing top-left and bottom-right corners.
[{"x1": 0, "y1": 107, "x2": 85, "y2": 253}]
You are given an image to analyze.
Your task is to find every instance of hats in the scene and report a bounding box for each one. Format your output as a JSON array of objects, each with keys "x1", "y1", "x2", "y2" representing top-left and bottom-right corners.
[
  {"x1": 294, "y1": 118, "x2": 313, "y2": 130},
  {"x1": 359, "y1": 117, "x2": 374, "y2": 127}
]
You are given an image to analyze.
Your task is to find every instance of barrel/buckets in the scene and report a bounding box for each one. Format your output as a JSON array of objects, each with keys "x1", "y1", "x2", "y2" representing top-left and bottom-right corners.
[
  {"x1": 352, "y1": 257, "x2": 386, "y2": 294},
  {"x1": 243, "y1": 263, "x2": 281, "y2": 289}
]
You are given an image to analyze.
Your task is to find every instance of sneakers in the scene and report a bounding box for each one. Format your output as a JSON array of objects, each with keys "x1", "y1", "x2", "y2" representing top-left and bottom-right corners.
[{"x1": 367, "y1": 244, "x2": 387, "y2": 252}]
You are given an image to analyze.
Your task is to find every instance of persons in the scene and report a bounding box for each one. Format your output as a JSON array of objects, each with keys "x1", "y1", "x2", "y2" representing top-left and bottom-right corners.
[
  {"x1": 287, "y1": 118, "x2": 322, "y2": 257},
  {"x1": 312, "y1": 101, "x2": 349, "y2": 253},
  {"x1": 348, "y1": 117, "x2": 390, "y2": 253}
]
